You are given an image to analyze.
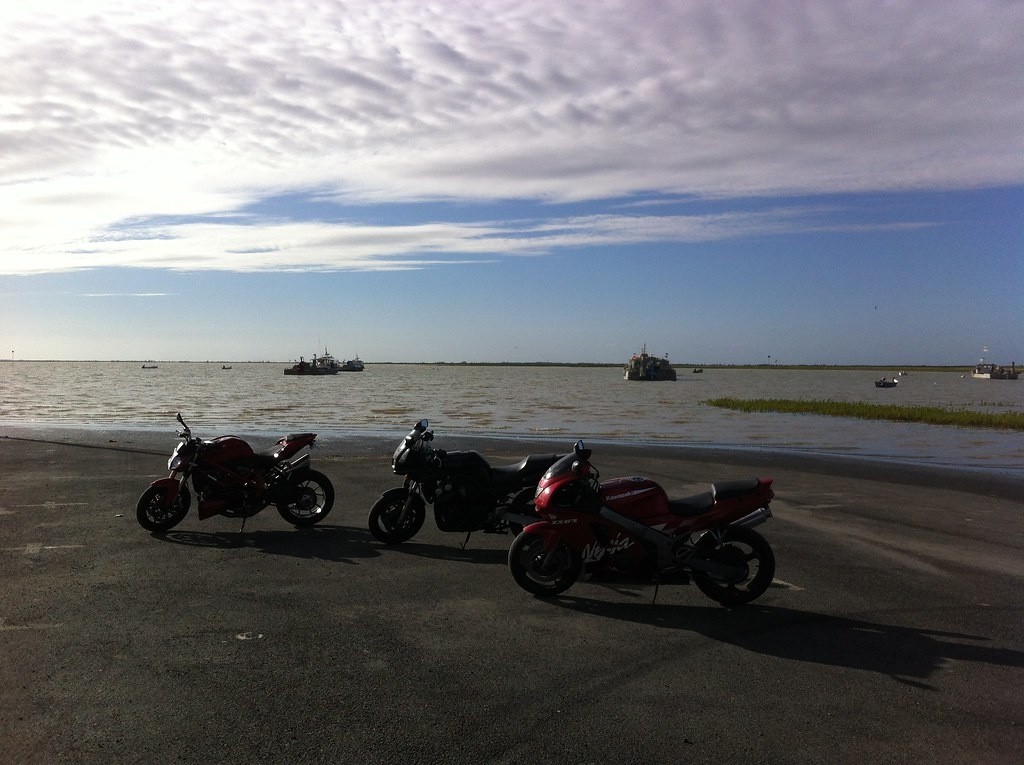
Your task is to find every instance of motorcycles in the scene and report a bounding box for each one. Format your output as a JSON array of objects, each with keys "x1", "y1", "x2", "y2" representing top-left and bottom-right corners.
[
  {"x1": 136, "y1": 413, "x2": 336, "y2": 532},
  {"x1": 366, "y1": 419, "x2": 568, "y2": 554},
  {"x1": 507, "y1": 439, "x2": 776, "y2": 607}
]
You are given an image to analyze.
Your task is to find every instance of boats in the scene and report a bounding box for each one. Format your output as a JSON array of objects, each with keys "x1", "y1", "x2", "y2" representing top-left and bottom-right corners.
[
  {"x1": 142, "y1": 366, "x2": 157, "y2": 368},
  {"x1": 336, "y1": 355, "x2": 365, "y2": 371},
  {"x1": 622, "y1": 344, "x2": 676, "y2": 381},
  {"x1": 692, "y1": 368, "x2": 703, "y2": 373},
  {"x1": 874, "y1": 380, "x2": 897, "y2": 388},
  {"x1": 899, "y1": 371, "x2": 907, "y2": 376},
  {"x1": 311, "y1": 349, "x2": 340, "y2": 374},
  {"x1": 222, "y1": 367, "x2": 232, "y2": 369},
  {"x1": 971, "y1": 362, "x2": 1019, "y2": 380},
  {"x1": 284, "y1": 354, "x2": 329, "y2": 374}
]
